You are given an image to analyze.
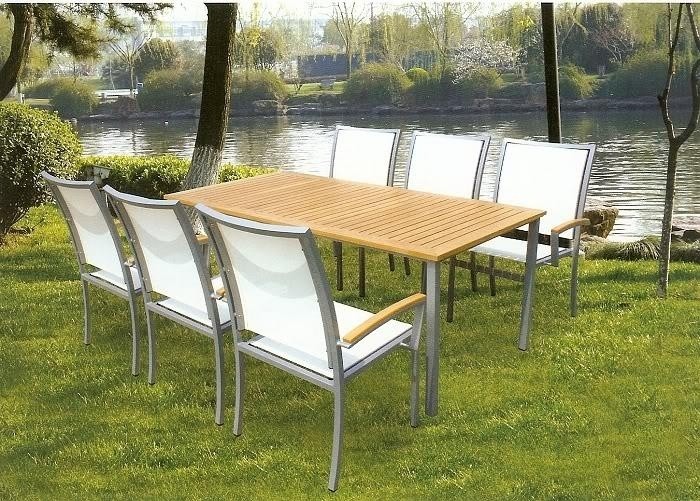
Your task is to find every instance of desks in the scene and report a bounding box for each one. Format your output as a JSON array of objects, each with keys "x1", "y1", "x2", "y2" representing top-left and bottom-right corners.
[{"x1": 161, "y1": 169, "x2": 547, "y2": 418}]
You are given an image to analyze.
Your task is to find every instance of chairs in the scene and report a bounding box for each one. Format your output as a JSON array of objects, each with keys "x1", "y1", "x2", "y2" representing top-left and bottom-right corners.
[
  {"x1": 326, "y1": 122, "x2": 597, "y2": 351},
  {"x1": 40, "y1": 170, "x2": 425, "y2": 492}
]
